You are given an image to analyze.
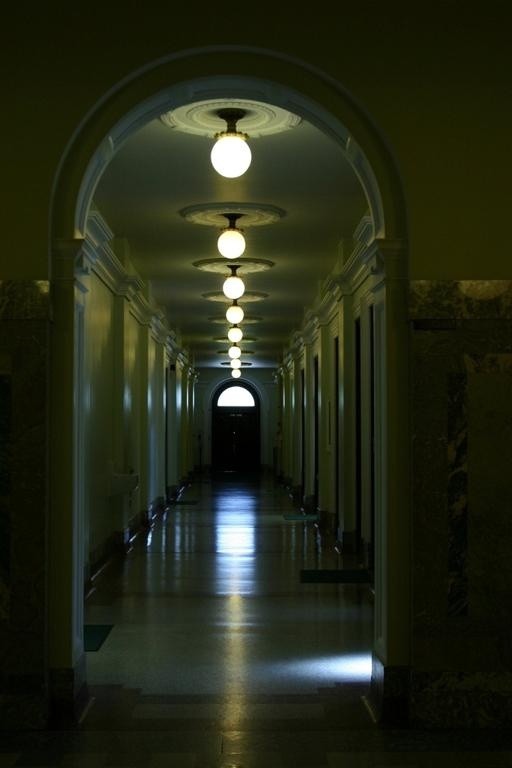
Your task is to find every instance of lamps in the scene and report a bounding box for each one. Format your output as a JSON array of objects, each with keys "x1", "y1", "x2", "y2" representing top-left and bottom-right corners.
[
  {"x1": 201, "y1": 290, "x2": 269, "y2": 325},
  {"x1": 190, "y1": 257, "x2": 275, "y2": 301},
  {"x1": 178, "y1": 201, "x2": 287, "y2": 259},
  {"x1": 207, "y1": 315, "x2": 263, "y2": 379},
  {"x1": 157, "y1": 99, "x2": 302, "y2": 179}
]
